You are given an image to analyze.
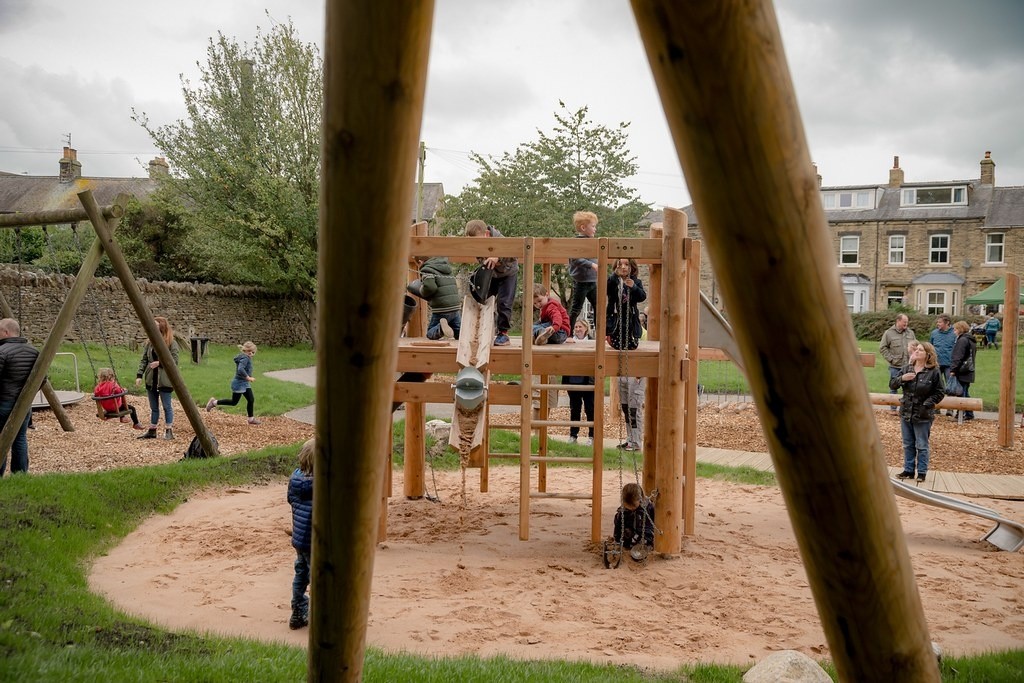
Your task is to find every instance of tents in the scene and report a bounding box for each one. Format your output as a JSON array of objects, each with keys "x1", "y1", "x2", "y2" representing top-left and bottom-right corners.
[{"x1": 964, "y1": 272, "x2": 1024, "y2": 315}]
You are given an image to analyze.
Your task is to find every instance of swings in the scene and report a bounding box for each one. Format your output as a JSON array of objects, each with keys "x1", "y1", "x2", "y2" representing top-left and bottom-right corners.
[{"x1": 42, "y1": 225, "x2": 132, "y2": 419}]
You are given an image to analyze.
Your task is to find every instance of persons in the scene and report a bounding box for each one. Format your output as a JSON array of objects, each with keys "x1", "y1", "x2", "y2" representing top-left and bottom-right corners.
[
  {"x1": 135, "y1": 317, "x2": 177, "y2": 441},
  {"x1": 400, "y1": 208, "x2": 652, "y2": 453},
  {"x1": 0, "y1": 317, "x2": 51, "y2": 476},
  {"x1": 613, "y1": 482, "x2": 656, "y2": 551},
  {"x1": 286, "y1": 437, "x2": 315, "y2": 629},
  {"x1": 206, "y1": 341, "x2": 262, "y2": 425},
  {"x1": 92, "y1": 367, "x2": 146, "y2": 430},
  {"x1": 879, "y1": 312, "x2": 1002, "y2": 482}
]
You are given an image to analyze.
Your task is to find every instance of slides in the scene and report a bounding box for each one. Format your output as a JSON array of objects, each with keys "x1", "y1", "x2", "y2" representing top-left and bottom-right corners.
[{"x1": 699, "y1": 292, "x2": 1024, "y2": 552}]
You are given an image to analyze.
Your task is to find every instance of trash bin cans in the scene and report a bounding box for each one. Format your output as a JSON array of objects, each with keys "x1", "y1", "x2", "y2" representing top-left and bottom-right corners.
[{"x1": 191, "y1": 336, "x2": 209, "y2": 363}]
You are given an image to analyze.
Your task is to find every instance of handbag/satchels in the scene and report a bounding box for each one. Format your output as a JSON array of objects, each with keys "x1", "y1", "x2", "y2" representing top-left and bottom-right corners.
[{"x1": 945, "y1": 375, "x2": 963, "y2": 395}]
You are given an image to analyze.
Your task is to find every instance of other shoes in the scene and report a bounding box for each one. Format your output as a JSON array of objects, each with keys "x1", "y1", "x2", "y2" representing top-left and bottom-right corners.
[
  {"x1": 566, "y1": 436, "x2": 578, "y2": 445},
  {"x1": 133, "y1": 422, "x2": 146, "y2": 430},
  {"x1": 120, "y1": 416, "x2": 130, "y2": 423},
  {"x1": 248, "y1": 418, "x2": 261, "y2": 426},
  {"x1": 625, "y1": 444, "x2": 639, "y2": 451},
  {"x1": 988, "y1": 344, "x2": 992, "y2": 349},
  {"x1": 206, "y1": 397, "x2": 216, "y2": 412},
  {"x1": 585, "y1": 436, "x2": 595, "y2": 447},
  {"x1": 163, "y1": 428, "x2": 174, "y2": 441},
  {"x1": 895, "y1": 470, "x2": 915, "y2": 479},
  {"x1": 440, "y1": 318, "x2": 454, "y2": 338},
  {"x1": 492, "y1": 332, "x2": 510, "y2": 346},
  {"x1": 137, "y1": 428, "x2": 157, "y2": 440},
  {"x1": 289, "y1": 619, "x2": 309, "y2": 630},
  {"x1": 945, "y1": 409, "x2": 953, "y2": 416},
  {"x1": 917, "y1": 473, "x2": 926, "y2": 482},
  {"x1": 995, "y1": 343, "x2": 1000, "y2": 350},
  {"x1": 535, "y1": 328, "x2": 554, "y2": 346},
  {"x1": 890, "y1": 410, "x2": 899, "y2": 416}
]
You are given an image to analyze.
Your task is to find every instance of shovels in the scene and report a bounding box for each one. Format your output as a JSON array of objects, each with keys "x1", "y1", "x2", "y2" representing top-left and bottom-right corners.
[
  {"x1": 469, "y1": 263, "x2": 494, "y2": 303},
  {"x1": 604, "y1": 541, "x2": 624, "y2": 568}
]
